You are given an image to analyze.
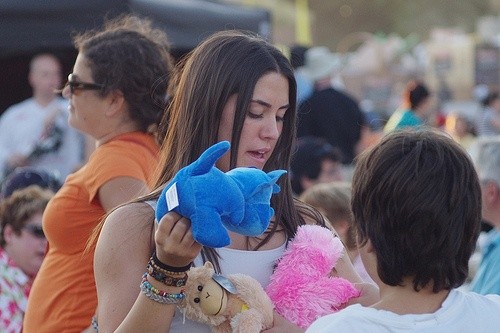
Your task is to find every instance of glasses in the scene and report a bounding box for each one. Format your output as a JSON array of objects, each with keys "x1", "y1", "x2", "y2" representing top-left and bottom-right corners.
[
  {"x1": 22, "y1": 223, "x2": 45, "y2": 237},
  {"x1": 66, "y1": 73, "x2": 104, "y2": 93}
]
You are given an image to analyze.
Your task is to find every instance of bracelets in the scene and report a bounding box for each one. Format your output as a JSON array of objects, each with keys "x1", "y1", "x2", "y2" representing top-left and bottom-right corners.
[
  {"x1": 140, "y1": 251, "x2": 193, "y2": 305},
  {"x1": 91, "y1": 316, "x2": 98, "y2": 332}
]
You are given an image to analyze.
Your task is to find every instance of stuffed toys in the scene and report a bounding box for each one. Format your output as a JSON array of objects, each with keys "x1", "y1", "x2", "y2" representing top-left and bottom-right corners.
[
  {"x1": 155, "y1": 141, "x2": 288, "y2": 248},
  {"x1": 176, "y1": 260, "x2": 274, "y2": 333},
  {"x1": 266, "y1": 224, "x2": 362, "y2": 331}
]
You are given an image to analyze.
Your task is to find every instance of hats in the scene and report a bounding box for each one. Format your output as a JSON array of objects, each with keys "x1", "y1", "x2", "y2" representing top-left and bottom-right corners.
[{"x1": 296, "y1": 45, "x2": 341, "y2": 80}]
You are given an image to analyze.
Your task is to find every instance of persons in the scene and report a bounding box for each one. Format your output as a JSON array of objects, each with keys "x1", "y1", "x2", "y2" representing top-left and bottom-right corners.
[
  {"x1": 92, "y1": 34, "x2": 380, "y2": 333},
  {"x1": 304, "y1": 130, "x2": 500, "y2": 333},
  {"x1": 0, "y1": 0, "x2": 500, "y2": 333}
]
